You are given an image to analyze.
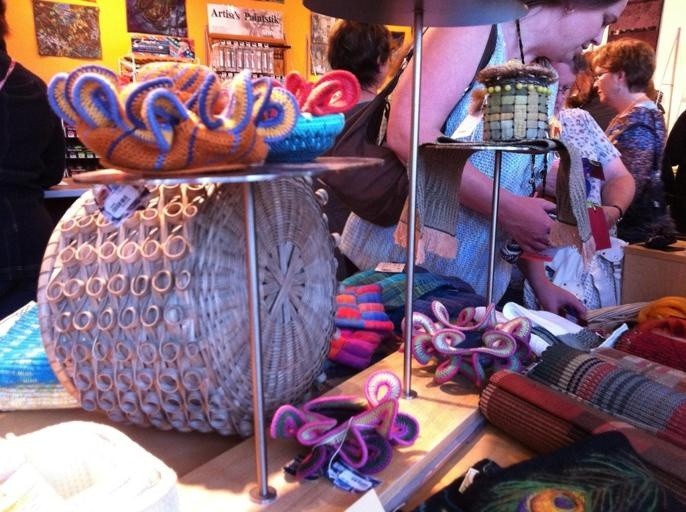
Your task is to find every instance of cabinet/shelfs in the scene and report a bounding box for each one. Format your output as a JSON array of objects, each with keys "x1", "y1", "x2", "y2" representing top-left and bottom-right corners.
[
  {"x1": 120, "y1": 36, "x2": 199, "y2": 85},
  {"x1": 205, "y1": 27, "x2": 291, "y2": 82}
]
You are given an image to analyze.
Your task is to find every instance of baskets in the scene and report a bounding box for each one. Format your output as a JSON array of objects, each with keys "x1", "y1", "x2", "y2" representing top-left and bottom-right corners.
[
  {"x1": 268, "y1": 115, "x2": 344, "y2": 166},
  {"x1": 478, "y1": 60, "x2": 553, "y2": 142},
  {"x1": 36, "y1": 176, "x2": 340, "y2": 438}
]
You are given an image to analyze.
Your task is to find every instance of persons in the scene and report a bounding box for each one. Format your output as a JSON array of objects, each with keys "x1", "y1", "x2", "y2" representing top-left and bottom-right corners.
[
  {"x1": 314, "y1": 18, "x2": 395, "y2": 232},
  {"x1": 0, "y1": 1, "x2": 71, "y2": 319},
  {"x1": 520, "y1": 58, "x2": 635, "y2": 313},
  {"x1": 335, "y1": 0, "x2": 628, "y2": 324},
  {"x1": 591, "y1": 38, "x2": 668, "y2": 225}
]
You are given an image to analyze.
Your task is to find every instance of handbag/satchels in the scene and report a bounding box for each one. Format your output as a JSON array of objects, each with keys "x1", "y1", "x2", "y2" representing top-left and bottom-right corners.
[
  {"x1": 317, "y1": 24, "x2": 498, "y2": 227},
  {"x1": 618, "y1": 121, "x2": 679, "y2": 251}
]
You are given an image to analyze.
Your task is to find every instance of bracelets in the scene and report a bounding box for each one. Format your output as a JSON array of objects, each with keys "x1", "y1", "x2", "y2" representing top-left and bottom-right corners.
[{"x1": 612, "y1": 203, "x2": 626, "y2": 224}]
